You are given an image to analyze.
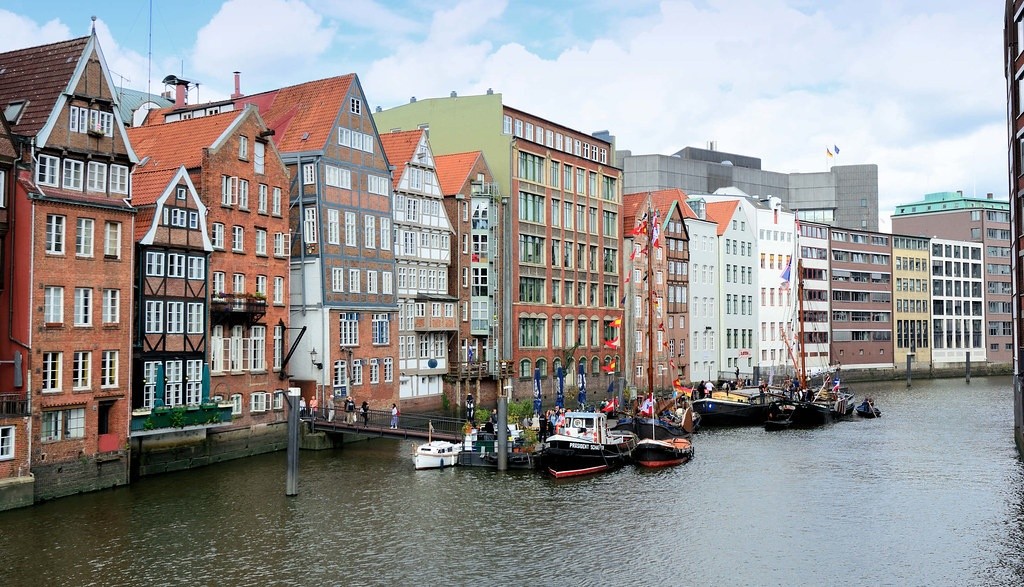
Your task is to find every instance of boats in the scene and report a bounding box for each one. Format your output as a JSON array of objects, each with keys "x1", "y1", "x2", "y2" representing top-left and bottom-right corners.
[
  {"x1": 544, "y1": 412, "x2": 638, "y2": 478},
  {"x1": 692, "y1": 384, "x2": 788, "y2": 426},
  {"x1": 856, "y1": 402, "x2": 880, "y2": 418},
  {"x1": 636, "y1": 393, "x2": 693, "y2": 468},
  {"x1": 413, "y1": 420, "x2": 461, "y2": 469}
]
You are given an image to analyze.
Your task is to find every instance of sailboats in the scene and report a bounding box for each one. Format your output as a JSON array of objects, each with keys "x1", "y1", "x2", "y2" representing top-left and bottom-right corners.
[
  {"x1": 765, "y1": 258, "x2": 854, "y2": 430},
  {"x1": 616, "y1": 206, "x2": 701, "y2": 437}
]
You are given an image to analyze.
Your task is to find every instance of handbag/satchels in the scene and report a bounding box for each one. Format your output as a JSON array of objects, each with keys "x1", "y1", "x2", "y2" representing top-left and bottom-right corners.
[
  {"x1": 396, "y1": 410, "x2": 400, "y2": 415},
  {"x1": 360, "y1": 407, "x2": 364, "y2": 414}
]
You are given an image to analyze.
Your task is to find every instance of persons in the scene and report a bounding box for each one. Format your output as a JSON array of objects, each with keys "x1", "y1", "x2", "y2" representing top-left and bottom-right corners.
[
  {"x1": 490, "y1": 409, "x2": 498, "y2": 423},
  {"x1": 299, "y1": 397, "x2": 306, "y2": 420},
  {"x1": 390, "y1": 404, "x2": 398, "y2": 430},
  {"x1": 481, "y1": 417, "x2": 494, "y2": 434},
  {"x1": 523, "y1": 405, "x2": 571, "y2": 443},
  {"x1": 735, "y1": 367, "x2": 739, "y2": 379},
  {"x1": 861, "y1": 397, "x2": 874, "y2": 412},
  {"x1": 601, "y1": 396, "x2": 618, "y2": 417},
  {"x1": 697, "y1": 380, "x2": 715, "y2": 399},
  {"x1": 759, "y1": 382, "x2": 766, "y2": 404},
  {"x1": 343, "y1": 396, "x2": 357, "y2": 425},
  {"x1": 783, "y1": 378, "x2": 815, "y2": 403},
  {"x1": 328, "y1": 395, "x2": 335, "y2": 422},
  {"x1": 360, "y1": 401, "x2": 370, "y2": 428},
  {"x1": 310, "y1": 396, "x2": 318, "y2": 420},
  {"x1": 721, "y1": 380, "x2": 730, "y2": 392},
  {"x1": 744, "y1": 376, "x2": 750, "y2": 386}
]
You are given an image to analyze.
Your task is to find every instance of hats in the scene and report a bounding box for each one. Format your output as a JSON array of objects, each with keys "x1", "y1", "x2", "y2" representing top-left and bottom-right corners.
[{"x1": 330, "y1": 394, "x2": 334, "y2": 397}]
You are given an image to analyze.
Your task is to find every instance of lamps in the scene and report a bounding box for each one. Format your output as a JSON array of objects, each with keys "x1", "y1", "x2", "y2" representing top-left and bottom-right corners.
[{"x1": 310, "y1": 346, "x2": 323, "y2": 369}]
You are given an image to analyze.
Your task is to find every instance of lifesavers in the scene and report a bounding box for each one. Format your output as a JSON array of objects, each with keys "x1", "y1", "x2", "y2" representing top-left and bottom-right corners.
[{"x1": 573, "y1": 419, "x2": 582, "y2": 427}]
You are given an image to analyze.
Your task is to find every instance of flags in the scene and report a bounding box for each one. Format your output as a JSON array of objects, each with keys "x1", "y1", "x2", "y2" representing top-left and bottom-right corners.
[
  {"x1": 640, "y1": 394, "x2": 653, "y2": 417},
  {"x1": 827, "y1": 149, "x2": 833, "y2": 158},
  {"x1": 832, "y1": 378, "x2": 840, "y2": 392},
  {"x1": 835, "y1": 146, "x2": 839, "y2": 154}
]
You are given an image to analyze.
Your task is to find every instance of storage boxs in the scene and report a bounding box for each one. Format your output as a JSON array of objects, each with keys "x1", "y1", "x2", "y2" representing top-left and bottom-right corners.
[{"x1": 475, "y1": 434, "x2": 514, "y2": 452}]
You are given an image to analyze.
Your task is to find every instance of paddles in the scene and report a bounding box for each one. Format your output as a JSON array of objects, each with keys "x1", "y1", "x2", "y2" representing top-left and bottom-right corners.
[{"x1": 867, "y1": 400, "x2": 878, "y2": 418}]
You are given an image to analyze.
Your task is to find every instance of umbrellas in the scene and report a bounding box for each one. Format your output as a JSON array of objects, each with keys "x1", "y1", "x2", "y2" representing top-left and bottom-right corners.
[
  {"x1": 468, "y1": 345, "x2": 473, "y2": 361},
  {"x1": 578, "y1": 364, "x2": 586, "y2": 409},
  {"x1": 533, "y1": 368, "x2": 542, "y2": 413},
  {"x1": 466, "y1": 393, "x2": 475, "y2": 427},
  {"x1": 555, "y1": 367, "x2": 564, "y2": 409}
]
